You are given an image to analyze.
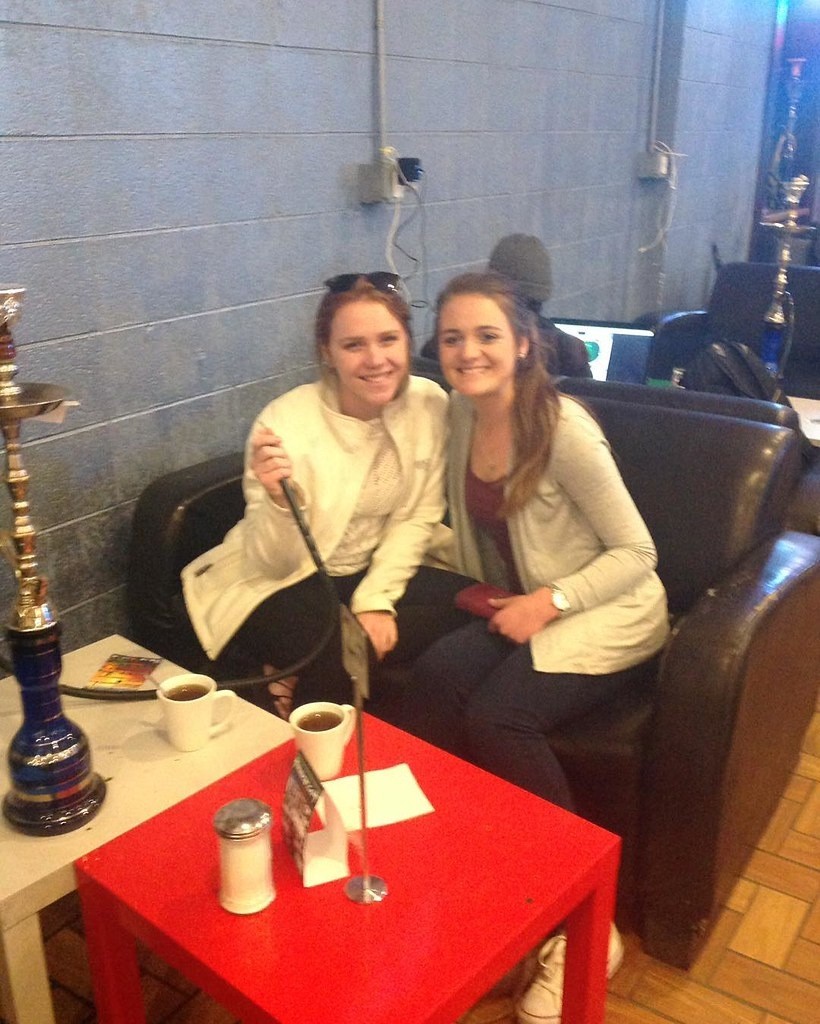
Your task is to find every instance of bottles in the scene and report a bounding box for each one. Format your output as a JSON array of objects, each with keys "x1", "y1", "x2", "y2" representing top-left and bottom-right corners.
[{"x1": 213, "y1": 796, "x2": 278, "y2": 916}]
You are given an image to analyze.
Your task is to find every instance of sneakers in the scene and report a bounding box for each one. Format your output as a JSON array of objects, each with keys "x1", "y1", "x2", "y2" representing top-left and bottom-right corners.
[{"x1": 514, "y1": 921, "x2": 624, "y2": 1024}]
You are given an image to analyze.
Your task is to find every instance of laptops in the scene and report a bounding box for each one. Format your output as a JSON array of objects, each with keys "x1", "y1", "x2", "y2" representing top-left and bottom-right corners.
[{"x1": 550, "y1": 317, "x2": 657, "y2": 384}]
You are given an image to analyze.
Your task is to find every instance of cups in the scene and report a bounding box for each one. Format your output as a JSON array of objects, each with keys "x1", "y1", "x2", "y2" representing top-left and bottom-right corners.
[
  {"x1": 156, "y1": 674, "x2": 237, "y2": 752},
  {"x1": 289, "y1": 701, "x2": 357, "y2": 781}
]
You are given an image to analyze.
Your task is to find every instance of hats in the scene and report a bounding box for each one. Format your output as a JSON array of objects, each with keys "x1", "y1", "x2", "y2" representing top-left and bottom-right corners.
[{"x1": 489, "y1": 235, "x2": 553, "y2": 302}]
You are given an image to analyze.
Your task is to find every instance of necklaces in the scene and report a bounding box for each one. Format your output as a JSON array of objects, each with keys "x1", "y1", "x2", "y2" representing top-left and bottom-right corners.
[{"x1": 475, "y1": 441, "x2": 499, "y2": 472}]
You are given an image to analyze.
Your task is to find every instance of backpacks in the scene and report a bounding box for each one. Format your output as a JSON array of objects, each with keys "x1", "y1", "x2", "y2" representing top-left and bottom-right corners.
[{"x1": 685, "y1": 344, "x2": 814, "y2": 472}]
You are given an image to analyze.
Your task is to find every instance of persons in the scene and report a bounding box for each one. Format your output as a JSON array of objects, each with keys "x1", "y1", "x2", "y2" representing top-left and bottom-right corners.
[
  {"x1": 407, "y1": 275, "x2": 672, "y2": 1024},
  {"x1": 181, "y1": 271, "x2": 449, "y2": 724},
  {"x1": 420, "y1": 235, "x2": 594, "y2": 382}
]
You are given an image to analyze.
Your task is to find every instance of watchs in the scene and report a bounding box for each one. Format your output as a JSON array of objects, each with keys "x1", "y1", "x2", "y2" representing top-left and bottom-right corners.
[{"x1": 544, "y1": 583, "x2": 573, "y2": 620}]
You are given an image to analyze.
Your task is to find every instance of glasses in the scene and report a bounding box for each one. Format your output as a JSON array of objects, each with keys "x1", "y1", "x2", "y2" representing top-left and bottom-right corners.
[{"x1": 324, "y1": 272, "x2": 402, "y2": 296}]
